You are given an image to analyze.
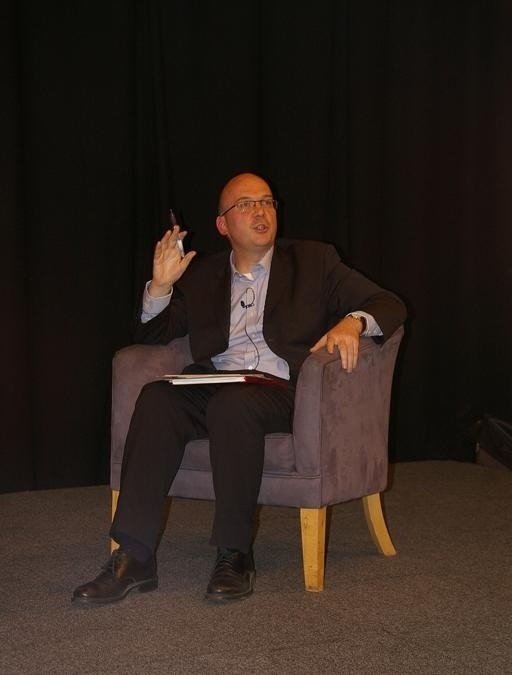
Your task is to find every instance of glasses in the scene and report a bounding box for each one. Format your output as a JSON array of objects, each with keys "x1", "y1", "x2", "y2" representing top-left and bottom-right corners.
[{"x1": 220, "y1": 199, "x2": 278, "y2": 218}]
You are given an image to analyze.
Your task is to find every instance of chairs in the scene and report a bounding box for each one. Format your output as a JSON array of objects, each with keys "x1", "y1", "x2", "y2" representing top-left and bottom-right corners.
[{"x1": 108, "y1": 324, "x2": 406, "y2": 593}]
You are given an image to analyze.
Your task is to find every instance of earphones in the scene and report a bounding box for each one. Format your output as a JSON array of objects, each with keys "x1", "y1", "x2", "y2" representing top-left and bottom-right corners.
[{"x1": 241, "y1": 300, "x2": 255, "y2": 308}]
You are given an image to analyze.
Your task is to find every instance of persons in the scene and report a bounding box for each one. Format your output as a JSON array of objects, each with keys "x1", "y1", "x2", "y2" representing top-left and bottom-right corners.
[{"x1": 74, "y1": 165, "x2": 408, "y2": 608}]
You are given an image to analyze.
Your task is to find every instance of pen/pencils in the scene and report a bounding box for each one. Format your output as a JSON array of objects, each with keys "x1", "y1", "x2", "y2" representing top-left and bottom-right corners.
[{"x1": 170, "y1": 208, "x2": 185, "y2": 259}]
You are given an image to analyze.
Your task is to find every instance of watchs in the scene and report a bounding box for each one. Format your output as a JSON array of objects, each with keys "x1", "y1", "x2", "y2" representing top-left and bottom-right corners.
[{"x1": 346, "y1": 312, "x2": 366, "y2": 334}]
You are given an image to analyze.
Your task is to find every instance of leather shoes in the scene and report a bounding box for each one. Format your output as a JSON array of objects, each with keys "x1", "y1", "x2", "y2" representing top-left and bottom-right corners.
[
  {"x1": 71, "y1": 545, "x2": 158, "y2": 604},
  {"x1": 206, "y1": 545, "x2": 256, "y2": 603}
]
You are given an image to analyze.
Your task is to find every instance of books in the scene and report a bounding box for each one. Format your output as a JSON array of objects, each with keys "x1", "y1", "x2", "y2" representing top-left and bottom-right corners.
[{"x1": 155, "y1": 372, "x2": 281, "y2": 386}]
